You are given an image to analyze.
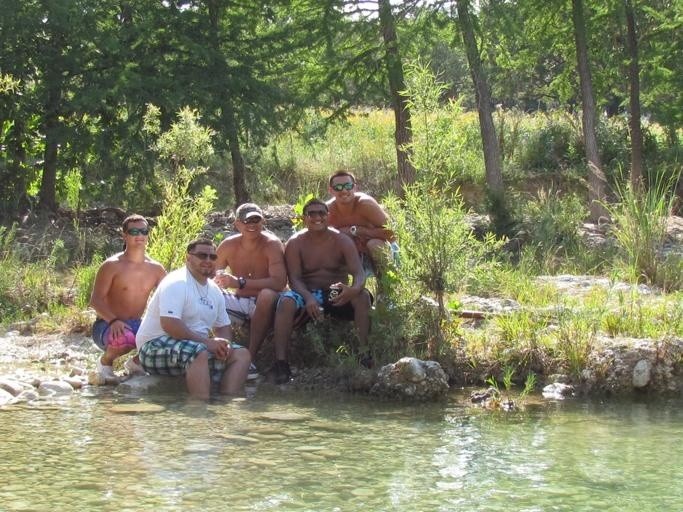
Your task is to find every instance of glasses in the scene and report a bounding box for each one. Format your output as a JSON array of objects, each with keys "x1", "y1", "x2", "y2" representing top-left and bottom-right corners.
[
  {"x1": 306, "y1": 210, "x2": 326, "y2": 217},
  {"x1": 128, "y1": 228, "x2": 148, "y2": 235},
  {"x1": 242, "y1": 217, "x2": 261, "y2": 224},
  {"x1": 189, "y1": 253, "x2": 217, "y2": 259},
  {"x1": 332, "y1": 183, "x2": 353, "y2": 191}
]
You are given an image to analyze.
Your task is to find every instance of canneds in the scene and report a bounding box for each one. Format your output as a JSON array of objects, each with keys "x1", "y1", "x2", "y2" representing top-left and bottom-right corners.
[
  {"x1": 328, "y1": 284, "x2": 342, "y2": 304},
  {"x1": 215, "y1": 269, "x2": 227, "y2": 289},
  {"x1": 314, "y1": 307, "x2": 324, "y2": 329}
]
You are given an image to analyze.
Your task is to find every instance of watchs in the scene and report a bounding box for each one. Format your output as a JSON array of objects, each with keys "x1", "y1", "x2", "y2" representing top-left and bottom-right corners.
[{"x1": 349, "y1": 225, "x2": 357, "y2": 237}]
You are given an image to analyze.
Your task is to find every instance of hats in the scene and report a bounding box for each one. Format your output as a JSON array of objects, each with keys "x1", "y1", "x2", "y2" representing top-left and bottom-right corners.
[{"x1": 236, "y1": 203, "x2": 264, "y2": 221}]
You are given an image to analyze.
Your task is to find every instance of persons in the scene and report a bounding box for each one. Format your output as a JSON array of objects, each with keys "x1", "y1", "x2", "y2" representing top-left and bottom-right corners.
[
  {"x1": 320, "y1": 170, "x2": 399, "y2": 300},
  {"x1": 267, "y1": 197, "x2": 376, "y2": 387},
  {"x1": 135, "y1": 239, "x2": 249, "y2": 399},
  {"x1": 206, "y1": 202, "x2": 285, "y2": 363},
  {"x1": 86, "y1": 214, "x2": 167, "y2": 377}
]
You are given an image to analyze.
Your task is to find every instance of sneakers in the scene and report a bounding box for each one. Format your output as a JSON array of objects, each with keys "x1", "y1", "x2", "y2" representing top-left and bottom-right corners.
[
  {"x1": 276, "y1": 360, "x2": 293, "y2": 386},
  {"x1": 212, "y1": 359, "x2": 227, "y2": 382},
  {"x1": 246, "y1": 363, "x2": 259, "y2": 380},
  {"x1": 97, "y1": 355, "x2": 147, "y2": 381},
  {"x1": 359, "y1": 355, "x2": 375, "y2": 369}
]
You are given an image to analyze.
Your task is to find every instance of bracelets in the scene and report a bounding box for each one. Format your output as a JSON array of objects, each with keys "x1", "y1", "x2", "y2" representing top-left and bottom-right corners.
[
  {"x1": 236, "y1": 277, "x2": 246, "y2": 289},
  {"x1": 106, "y1": 317, "x2": 121, "y2": 326}
]
